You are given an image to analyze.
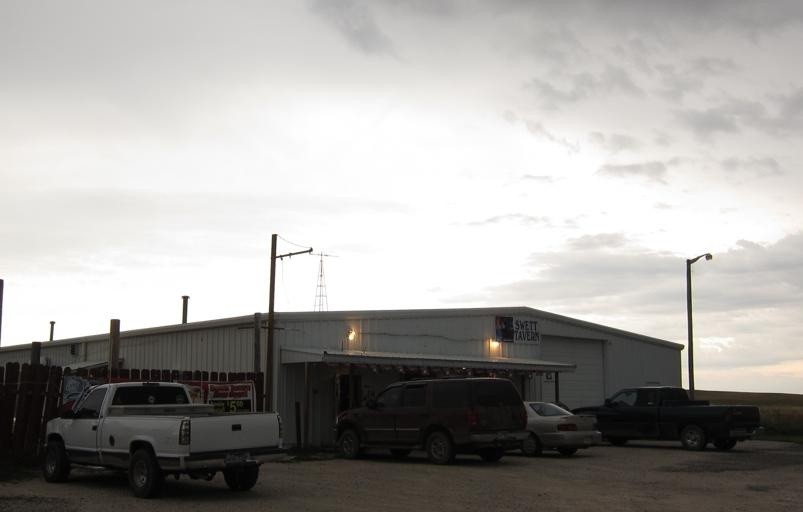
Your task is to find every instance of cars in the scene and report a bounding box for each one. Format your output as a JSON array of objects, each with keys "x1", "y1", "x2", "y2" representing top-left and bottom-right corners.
[{"x1": 334, "y1": 377, "x2": 601, "y2": 465}]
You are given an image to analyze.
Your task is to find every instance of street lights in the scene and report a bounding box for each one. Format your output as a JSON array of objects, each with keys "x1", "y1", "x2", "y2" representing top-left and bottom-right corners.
[
  {"x1": 686, "y1": 253, "x2": 713, "y2": 400},
  {"x1": 266, "y1": 234, "x2": 314, "y2": 412}
]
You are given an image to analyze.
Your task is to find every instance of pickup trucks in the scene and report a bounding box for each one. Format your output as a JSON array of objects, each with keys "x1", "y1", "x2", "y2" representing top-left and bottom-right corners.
[
  {"x1": 43, "y1": 381, "x2": 284, "y2": 498},
  {"x1": 569, "y1": 386, "x2": 761, "y2": 451}
]
